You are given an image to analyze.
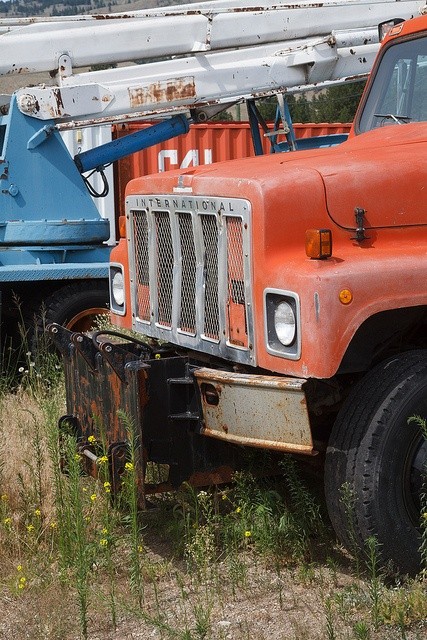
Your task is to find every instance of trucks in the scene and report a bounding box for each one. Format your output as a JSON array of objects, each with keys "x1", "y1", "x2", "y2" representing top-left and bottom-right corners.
[
  {"x1": 46, "y1": 14, "x2": 427, "y2": 577},
  {"x1": 52, "y1": 120, "x2": 354, "y2": 239},
  {"x1": 0, "y1": 0, "x2": 426, "y2": 386}
]
